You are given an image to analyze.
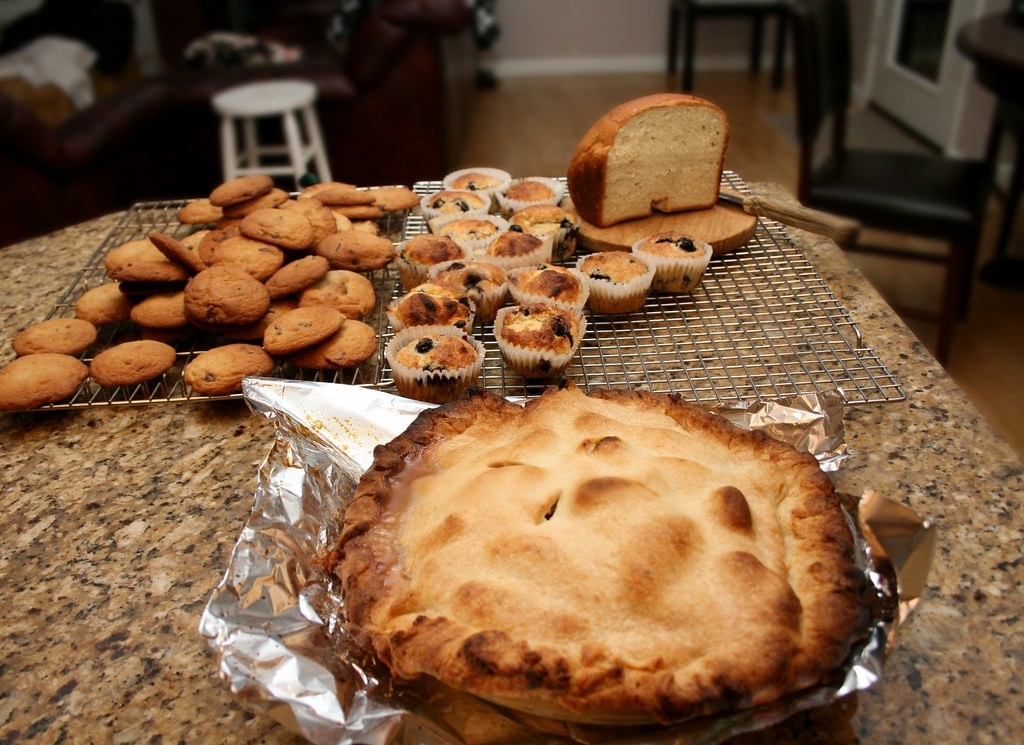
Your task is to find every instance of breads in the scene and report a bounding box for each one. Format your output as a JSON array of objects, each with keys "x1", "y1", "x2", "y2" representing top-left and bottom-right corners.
[
  {"x1": 332, "y1": 375, "x2": 862, "y2": 725},
  {"x1": 566, "y1": 92, "x2": 730, "y2": 229}
]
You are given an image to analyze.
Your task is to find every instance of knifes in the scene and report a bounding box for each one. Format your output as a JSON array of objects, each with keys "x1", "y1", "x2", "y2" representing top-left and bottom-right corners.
[{"x1": 719, "y1": 192, "x2": 859, "y2": 242}]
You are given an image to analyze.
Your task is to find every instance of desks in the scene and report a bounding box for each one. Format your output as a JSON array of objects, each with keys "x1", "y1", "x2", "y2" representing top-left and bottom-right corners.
[{"x1": 958, "y1": 15, "x2": 1024, "y2": 290}]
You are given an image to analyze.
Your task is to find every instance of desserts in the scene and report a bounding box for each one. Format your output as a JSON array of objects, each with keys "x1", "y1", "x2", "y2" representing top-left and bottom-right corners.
[{"x1": 386, "y1": 166, "x2": 713, "y2": 404}]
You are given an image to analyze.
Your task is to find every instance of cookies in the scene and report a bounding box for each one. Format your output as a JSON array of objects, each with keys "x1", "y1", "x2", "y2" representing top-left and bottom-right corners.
[{"x1": 0, "y1": 172, "x2": 420, "y2": 411}]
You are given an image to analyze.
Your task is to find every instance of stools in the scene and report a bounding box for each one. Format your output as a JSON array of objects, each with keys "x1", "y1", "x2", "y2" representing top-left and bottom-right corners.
[{"x1": 212, "y1": 78, "x2": 334, "y2": 193}]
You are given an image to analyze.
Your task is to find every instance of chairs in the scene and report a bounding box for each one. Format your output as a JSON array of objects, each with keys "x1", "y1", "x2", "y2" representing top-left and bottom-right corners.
[
  {"x1": 788, "y1": 1, "x2": 989, "y2": 369},
  {"x1": 663, "y1": 1, "x2": 791, "y2": 94}
]
